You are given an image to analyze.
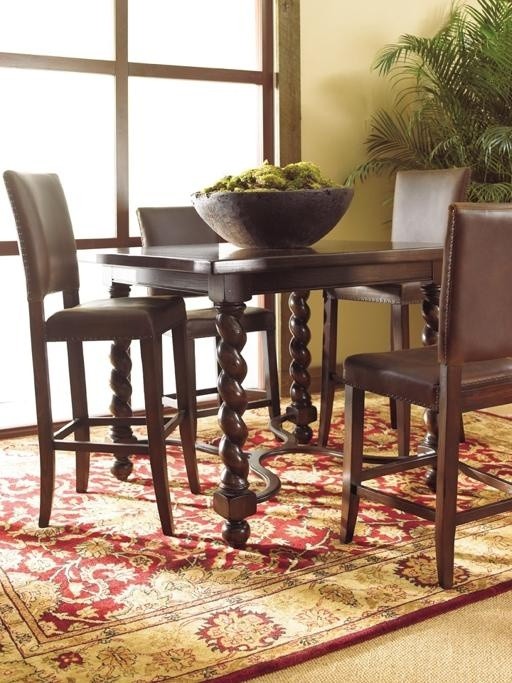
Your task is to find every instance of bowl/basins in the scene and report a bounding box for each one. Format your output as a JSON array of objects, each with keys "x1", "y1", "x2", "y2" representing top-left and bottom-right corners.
[{"x1": 189, "y1": 187, "x2": 354, "y2": 248}]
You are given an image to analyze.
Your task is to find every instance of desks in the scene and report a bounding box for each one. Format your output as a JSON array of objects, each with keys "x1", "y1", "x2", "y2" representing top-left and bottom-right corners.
[{"x1": 95, "y1": 238, "x2": 444, "y2": 549}]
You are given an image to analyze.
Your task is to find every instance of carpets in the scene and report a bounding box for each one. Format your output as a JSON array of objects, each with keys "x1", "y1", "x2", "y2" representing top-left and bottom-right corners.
[{"x1": 0, "y1": 383, "x2": 512, "y2": 683}]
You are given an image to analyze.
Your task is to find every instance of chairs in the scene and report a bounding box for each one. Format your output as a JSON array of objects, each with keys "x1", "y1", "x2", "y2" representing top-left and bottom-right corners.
[
  {"x1": 134, "y1": 204, "x2": 286, "y2": 443},
  {"x1": 0, "y1": 167, "x2": 203, "y2": 537},
  {"x1": 316, "y1": 168, "x2": 474, "y2": 452},
  {"x1": 339, "y1": 200, "x2": 511, "y2": 589}
]
